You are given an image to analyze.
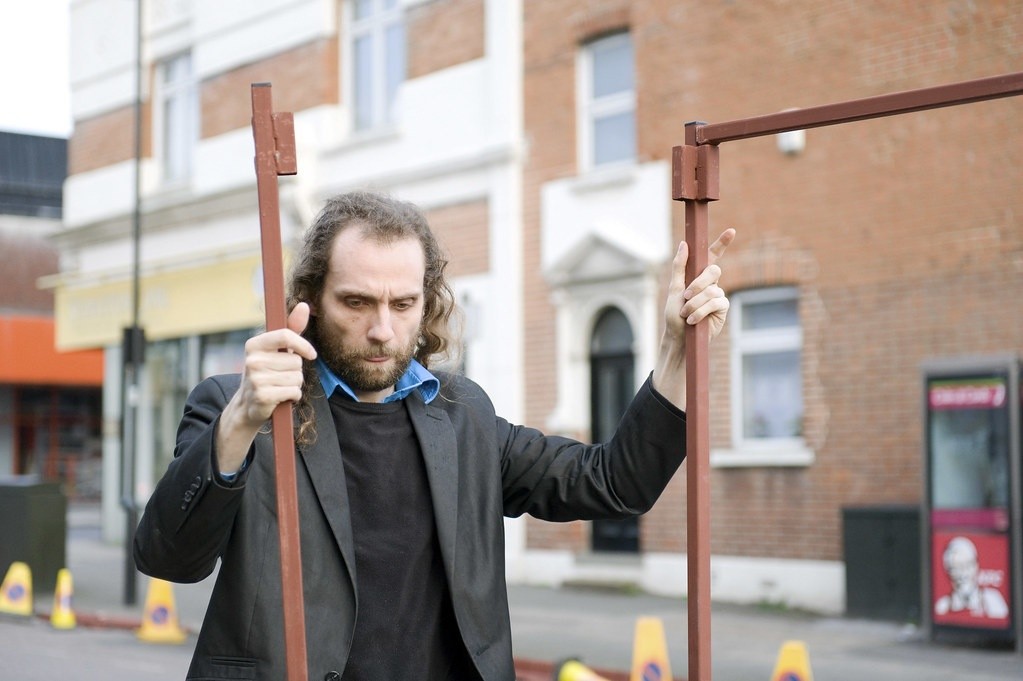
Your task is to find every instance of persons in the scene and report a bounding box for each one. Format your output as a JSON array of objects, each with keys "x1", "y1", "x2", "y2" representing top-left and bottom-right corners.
[{"x1": 132, "y1": 189, "x2": 738, "y2": 681}]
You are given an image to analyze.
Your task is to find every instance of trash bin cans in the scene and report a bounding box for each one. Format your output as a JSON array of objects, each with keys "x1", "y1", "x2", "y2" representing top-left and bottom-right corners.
[{"x1": 1, "y1": 474, "x2": 68, "y2": 592}]
